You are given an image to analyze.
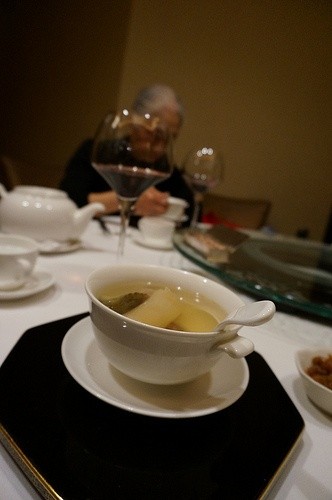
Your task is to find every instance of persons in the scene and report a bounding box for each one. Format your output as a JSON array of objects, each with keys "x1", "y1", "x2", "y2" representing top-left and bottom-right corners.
[{"x1": 58, "y1": 80, "x2": 203, "y2": 231}]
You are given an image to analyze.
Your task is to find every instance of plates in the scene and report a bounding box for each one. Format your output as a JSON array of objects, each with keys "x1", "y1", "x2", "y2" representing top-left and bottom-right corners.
[
  {"x1": 133, "y1": 236, "x2": 175, "y2": 252},
  {"x1": 60, "y1": 314, "x2": 250, "y2": 418},
  {"x1": 3, "y1": 266, "x2": 57, "y2": 301}
]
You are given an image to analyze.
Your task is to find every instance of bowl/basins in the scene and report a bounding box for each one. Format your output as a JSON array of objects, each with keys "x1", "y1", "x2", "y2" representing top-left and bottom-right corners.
[{"x1": 295, "y1": 346, "x2": 332, "y2": 419}]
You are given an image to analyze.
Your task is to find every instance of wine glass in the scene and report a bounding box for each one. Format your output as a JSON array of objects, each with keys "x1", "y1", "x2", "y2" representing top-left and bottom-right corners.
[
  {"x1": 92, "y1": 108, "x2": 177, "y2": 264},
  {"x1": 182, "y1": 141, "x2": 220, "y2": 235}
]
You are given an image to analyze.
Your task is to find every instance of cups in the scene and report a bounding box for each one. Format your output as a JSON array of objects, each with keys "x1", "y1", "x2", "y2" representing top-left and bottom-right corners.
[
  {"x1": 137, "y1": 217, "x2": 172, "y2": 243},
  {"x1": 1, "y1": 231, "x2": 38, "y2": 290},
  {"x1": 167, "y1": 196, "x2": 185, "y2": 218},
  {"x1": 86, "y1": 264, "x2": 268, "y2": 390}
]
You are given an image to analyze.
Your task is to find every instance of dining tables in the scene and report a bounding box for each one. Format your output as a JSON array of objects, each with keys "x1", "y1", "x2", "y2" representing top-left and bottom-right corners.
[{"x1": 0, "y1": 224, "x2": 331, "y2": 500}]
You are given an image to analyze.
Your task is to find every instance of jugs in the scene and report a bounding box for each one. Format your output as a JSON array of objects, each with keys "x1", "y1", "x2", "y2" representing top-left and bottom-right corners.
[{"x1": 1, "y1": 185, "x2": 108, "y2": 243}]
